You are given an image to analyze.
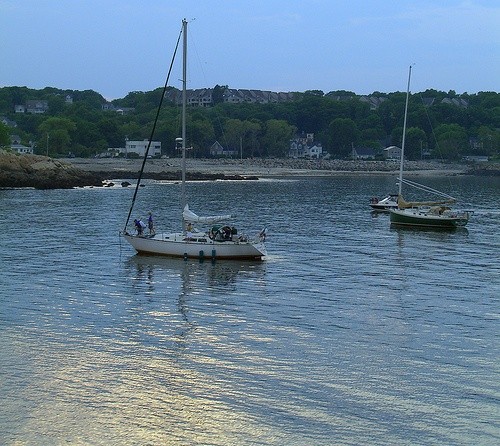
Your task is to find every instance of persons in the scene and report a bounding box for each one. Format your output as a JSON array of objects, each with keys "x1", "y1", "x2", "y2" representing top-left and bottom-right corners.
[
  {"x1": 370, "y1": 197, "x2": 378, "y2": 204},
  {"x1": 133, "y1": 219, "x2": 146, "y2": 235},
  {"x1": 187, "y1": 223, "x2": 193, "y2": 243},
  {"x1": 223, "y1": 227, "x2": 237, "y2": 238},
  {"x1": 148, "y1": 212, "x2": 155, "y2": 234}
]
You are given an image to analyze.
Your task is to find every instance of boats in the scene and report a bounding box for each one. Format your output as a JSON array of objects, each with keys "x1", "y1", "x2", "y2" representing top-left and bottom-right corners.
[{"x1": 369, "y1": 195, "x2": 398, "y2": 212}]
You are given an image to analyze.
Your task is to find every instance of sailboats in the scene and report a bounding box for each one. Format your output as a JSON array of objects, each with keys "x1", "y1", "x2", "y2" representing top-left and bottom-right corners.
[
  {"x1": 388, "y1": 64, "x2": 472, "y2": 229},
  {"x1": 120, "y1": 17, "x2": 268, "y2": 261}
]
